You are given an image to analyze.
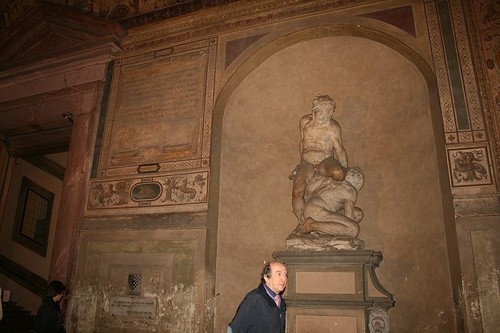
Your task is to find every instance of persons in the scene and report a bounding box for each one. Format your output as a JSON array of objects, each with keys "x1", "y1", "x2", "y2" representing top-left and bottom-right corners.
[
  {"x1": 36, "y1": 280, "x2": 64, "y2": 333},
  {"x1": 300, "y1": 167, "x2": 366, "y2": 238},
  {"x1": 226, "y1": 260, "x2": 288, "y2": 333},
  {"x1": 288, "y1": 95, "x2": 350, "y2": 235}
]
